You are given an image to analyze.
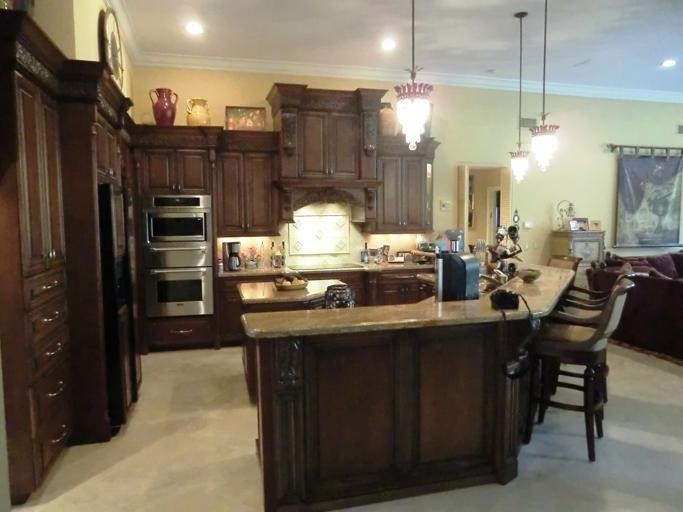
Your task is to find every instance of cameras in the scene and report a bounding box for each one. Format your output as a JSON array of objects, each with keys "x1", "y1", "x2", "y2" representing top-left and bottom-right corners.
[{"x1": 490, "y1": 289, "x2": 519, "y2": 310}]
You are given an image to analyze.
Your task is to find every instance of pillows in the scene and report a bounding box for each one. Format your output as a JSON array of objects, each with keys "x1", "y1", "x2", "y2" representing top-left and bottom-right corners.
[{"x1": 604, "y1": 253, "x2": 683, "y2": 280}]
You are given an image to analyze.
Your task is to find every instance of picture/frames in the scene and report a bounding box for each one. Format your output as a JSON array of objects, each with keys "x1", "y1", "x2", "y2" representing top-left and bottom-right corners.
[{"x1": 567, "y1": 217, "x2": 589, "y2": 230}]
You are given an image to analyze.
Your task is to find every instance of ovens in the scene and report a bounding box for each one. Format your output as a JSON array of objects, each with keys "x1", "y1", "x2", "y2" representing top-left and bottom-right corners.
[{"x1": 142, "y1": 194, "x2": 214, "y2": 318}]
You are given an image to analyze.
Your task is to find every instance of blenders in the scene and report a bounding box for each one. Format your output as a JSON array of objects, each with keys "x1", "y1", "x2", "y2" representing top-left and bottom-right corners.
[{"x1": 227, "y1": 242, "x2": 242, "y2": 271}]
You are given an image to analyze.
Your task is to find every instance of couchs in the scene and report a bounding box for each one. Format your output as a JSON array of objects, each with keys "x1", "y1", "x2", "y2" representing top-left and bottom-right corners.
[{"x1": 597, "y1": 250, "x2": 683, "y2": 360}]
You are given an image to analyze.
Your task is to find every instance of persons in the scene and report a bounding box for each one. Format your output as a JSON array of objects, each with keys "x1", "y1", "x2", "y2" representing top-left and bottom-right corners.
[{"x1": 635, "y1": 196, "x2": 679, "y2": 244}]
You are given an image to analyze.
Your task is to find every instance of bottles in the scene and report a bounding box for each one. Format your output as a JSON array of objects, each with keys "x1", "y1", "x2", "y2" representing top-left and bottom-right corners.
[
  {"x1": 434, "y1": 246, "x2": 439, "y2": 254},
  {"x1": 477, "y1": 261, "x2": 486, "y2": 275},
  {"x1": 216, "y1": 259, "x2": 224, "y2": 273},
  {"x1": 364, "y1": 242, "x2": 369, "y2": 263},
  {"x1": 185, "y1": 99, "x2": 211, "y2": 127},
  {"x1": 379, "y1": 104, "x2": 395, "y2": 137},
  {"x1": 269, "y1": 241, "x2": 285, "y2": 268},
  {"x1": 495, "y1": 225, "x2": 519, "y2": 244},
  {"x1": 148, "y1": 88, "x2": 180, "y2": 127}
]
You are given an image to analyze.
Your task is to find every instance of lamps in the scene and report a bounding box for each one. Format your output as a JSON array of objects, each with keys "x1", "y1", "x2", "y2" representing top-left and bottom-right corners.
[
  {"x1": 393, "y1": 1, "x2": 435, "y2": 149},
  {"x1": 444, "y1": 228, "x2": 464, "y2": 253},
  {"x1": 530, "y1": 0, "x2": 559, "y2": 172},
  {"x1": 508, "y1": 12, "x2": 534, "y2": 183}
]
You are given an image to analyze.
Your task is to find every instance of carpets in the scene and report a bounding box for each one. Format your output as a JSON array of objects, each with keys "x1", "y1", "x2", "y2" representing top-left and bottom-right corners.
[{"x1": 608, "y1": 337, "x2": 683, "y2": 365}]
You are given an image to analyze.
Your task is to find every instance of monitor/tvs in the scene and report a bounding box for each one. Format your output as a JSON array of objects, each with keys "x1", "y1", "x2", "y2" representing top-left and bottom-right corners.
[{"x1": 435, "y1": 251, "x2": 480, "y2": 301}]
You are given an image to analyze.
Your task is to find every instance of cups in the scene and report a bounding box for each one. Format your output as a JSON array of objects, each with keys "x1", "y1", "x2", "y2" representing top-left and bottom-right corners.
[{"x1": 451, "y1": 240, "x2": 459, "y2": 253}]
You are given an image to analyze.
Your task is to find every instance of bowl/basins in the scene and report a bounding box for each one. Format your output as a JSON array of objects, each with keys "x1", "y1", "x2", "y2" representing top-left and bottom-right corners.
[
  {"x1": 516, "y1": 270, "x2": 542, "y2": 283},
  {"x1": 274, "y1": 275, "x2": 307, "y2": 291}
]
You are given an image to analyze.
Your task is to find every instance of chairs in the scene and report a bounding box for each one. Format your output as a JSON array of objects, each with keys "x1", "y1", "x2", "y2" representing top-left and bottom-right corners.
[{"x1": 521, "y1": 255, "x2": 633, "y2": 462}]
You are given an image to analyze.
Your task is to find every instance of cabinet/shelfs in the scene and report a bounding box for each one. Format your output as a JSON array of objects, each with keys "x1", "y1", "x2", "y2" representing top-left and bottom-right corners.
[
  {"x1": 550, "y1": 231, "x2": 605, "y2": 286},
  {"x1": 0, "y1": 8, "x2": 77, "y2": 507}
]
